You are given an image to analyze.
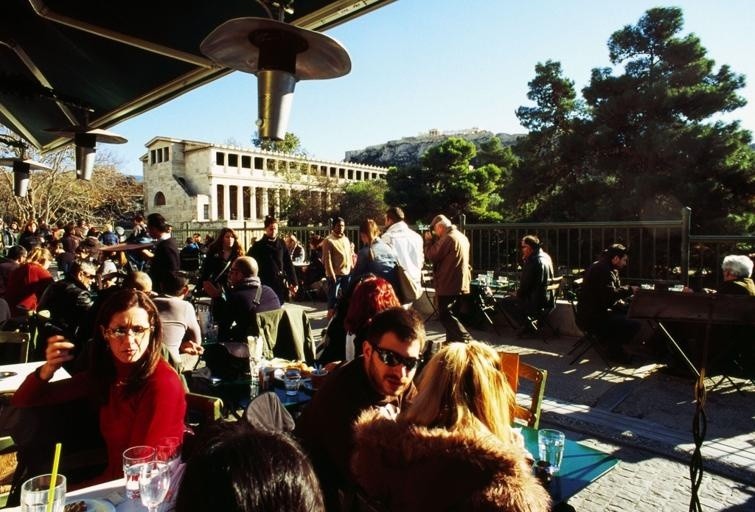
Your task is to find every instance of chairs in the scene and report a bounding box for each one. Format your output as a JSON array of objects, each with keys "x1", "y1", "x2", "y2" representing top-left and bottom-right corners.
[
  {"x1": 252, "y1": 305, "x2": 316, "y2": 362},
  {"x1": 0, "y1": 331, "x2": 31, "y2": 366},
  {"x1": 508, "y1": 276, "x2": 564, "y2": 345},
  {"x1": 420, "y1": 269, "x2": 461, "y2": 326},
  {"x1": 563, "y1": 288, "x2": 619, "y2": 371},
  {"x1": 496, "y1": 351, "x2": 550, "y2": 430}
]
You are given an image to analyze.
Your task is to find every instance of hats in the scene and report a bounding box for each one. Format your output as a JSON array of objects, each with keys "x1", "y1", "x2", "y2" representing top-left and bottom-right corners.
[{"x1": 430, "y1": 215, "x2": 444, "y2": 228}]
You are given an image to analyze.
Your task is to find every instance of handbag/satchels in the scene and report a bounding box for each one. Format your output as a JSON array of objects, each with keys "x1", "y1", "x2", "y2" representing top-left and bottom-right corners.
[
  {"x1": 272, "y1": 262, "x2": 292, "y2": 303},
  {"x1": 386, "y1": 242, "x2": 417, "y2": 304}
]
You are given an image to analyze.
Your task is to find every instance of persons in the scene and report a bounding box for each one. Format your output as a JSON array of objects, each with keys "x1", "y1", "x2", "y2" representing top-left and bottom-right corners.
[
  {"x1": 142, "y1": 212, "x2": 181, "y2": 291},
  {"x1": 322, "y1": 217, "x2": 351, "y2": 308},
  {"x1": 37, "y1": 259, "x2": 97, "y2": 332},
  {"x1": 15, "y1": 247, "x2": 52, "y2": 312},
  {"x1": 0, "y1": 245, "x2": 28, "y2": 327},
  {"x1": 344, "y1": 276, "x2": 402, "y2": 352},
  {"x1": 577, "y1": 242, "x2": 641, "y2": 362},
  {"x1": 350, "y1": 218, "x2": 404, "y2": 305},
  {"x1": 165, "y1": 224, "x2": 174, "y2": 233},
  {"x1": 203, "y1": 255, "x2": 281, "y2": 343},
  {"x1": 423, "y1": 214, "x2": 475, "y2": 343},
  {"x1": 11, "y1": 289, "x2": 188, "y2": 495},
  {"x1": 1, "y1": 210, "x2": 147, "y2": 258},
  {"x1": 173, "y1": 426, "x2": 327, "y2": 512},
  {"x1": 285, "y1": 234, "x2": 325, "y2": 296},
  {"x1": 297, "y1": 306, "x2": 427, "y2": 512},
  {"x1": 381, "y1": 206, "x2": 424, "y2": 301},
  {"x1": 354, "y1": 342, "x2": 576, "y2": 512},
  {"x1": 350, "y1": 240, "x2": 359, "y2": 270},
  {"x1": 194, "y1": 227, "x2": 243, "y2": 298},
  {"x1": 716, "y1": 254, "x2": 755, "y2": 295},
  {"x1": 193, "y1": 233, "x2": 204, "y2": 252},
  {"x1": 122, "y1": 270, "x2": 153, "y2": 297},
  {"x1": 152, "y1": 271, "x2": 201, "y2": 374},
  {"x1": 180, "y1": 237, "x2": 200, "y2": 272},
  {"x1": 247, "y1": 216, "x2": 299, "y2": 306},
  {"x1": 200, "y1": 233, "x2": 216, "y2": 254},
  {"x1": 502, "y1": 235, "x2": 556, "y2": 340}
]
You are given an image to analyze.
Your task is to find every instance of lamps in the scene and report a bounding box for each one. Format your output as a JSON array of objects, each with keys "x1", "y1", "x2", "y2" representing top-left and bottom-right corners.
[
  {"x1": 197, "y1": 1, "x2": 353, "y2": 142},
  {"x1": 0, "y1": 137, "x2": 51, "y2": 197},
  {"x1": 40, "y1": 97, "x2": 131, "y2": 184}
]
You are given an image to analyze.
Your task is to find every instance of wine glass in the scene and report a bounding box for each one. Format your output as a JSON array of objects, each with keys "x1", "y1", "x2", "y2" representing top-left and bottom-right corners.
[{"x1": 138, "y1": 462, "x2": 170, "y2": 511}]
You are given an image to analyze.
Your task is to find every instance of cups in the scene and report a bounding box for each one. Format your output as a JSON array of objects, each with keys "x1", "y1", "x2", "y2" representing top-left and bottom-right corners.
[
  {"x1": 20, "y1": 473, "x2": 66, "y2": 512},
  {"x1": 538, "y1": 428, "x2": 565, "y2": 473},
  {"x1": 156, "y1": 436, "x2": 182, "y2": 463},
  {"x1": 310, "y1": 368, "x2": 328, "y2": 389},
  {"x1": 284, "y1": 371, "x2": 302, "y2": 396},
  {"x1": 486, "y1": 270, "x2": 494, "y2": 283},
  {"x1": 500, "y1": 276, "x2": 508, "y2": 281},
  {"x1": 123, "y1": 445, "x2": 156, "y2": 501}
]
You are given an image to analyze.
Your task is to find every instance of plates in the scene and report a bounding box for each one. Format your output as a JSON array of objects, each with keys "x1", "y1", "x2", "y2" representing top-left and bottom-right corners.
[
  {"x1": 66, "y1": 498, "x2": 118, "y2": 512},
  {"x1": 274, "y1": 368, "x2": 317, "y2": 382},
  {"x1": 0, "y1": 371, "x2": 18, "y2": 379},
  {"x1": 496, "y1": 280, "x2": 509, "y2": 284}
]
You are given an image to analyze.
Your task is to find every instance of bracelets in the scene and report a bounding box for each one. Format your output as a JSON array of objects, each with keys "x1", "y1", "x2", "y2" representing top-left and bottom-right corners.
[{"x1": 34, "y1": 365, "x2": 52, "y2": 382}]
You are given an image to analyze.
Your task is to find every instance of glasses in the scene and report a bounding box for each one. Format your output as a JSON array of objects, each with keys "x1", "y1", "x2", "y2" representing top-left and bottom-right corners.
[
  {"x1": 228, "y1": 267, "x2": 239, "y2": 272},
  {"x1": 107, "y1": 325, "x2": 151, "y2": 336},
  {"x1": 85, "y1": 275, "x2": 96, "y2": 279},
  {"x1": 372, "y1": 345, "x2": 424, "y2": 369}
]
